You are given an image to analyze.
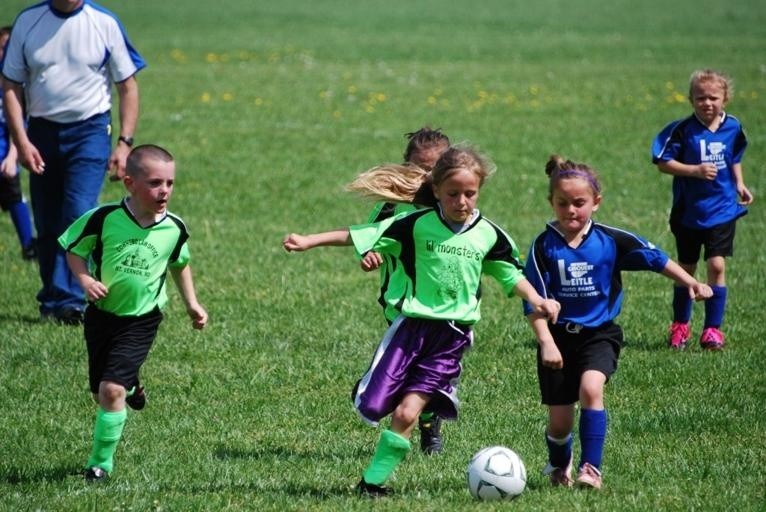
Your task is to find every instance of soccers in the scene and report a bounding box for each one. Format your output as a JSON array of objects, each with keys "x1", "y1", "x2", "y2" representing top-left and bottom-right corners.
[{"x1": 468, "y1": 447, "x2": 527, "y2": 502}]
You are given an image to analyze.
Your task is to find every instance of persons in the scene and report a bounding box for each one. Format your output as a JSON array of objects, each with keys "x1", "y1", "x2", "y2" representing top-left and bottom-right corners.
[
  {"x1": 354, "y1": 126, "x2": 459, "y2": 454},
  {"x1": 284, "y1": 140, "x2": 561, "y2": 499},
  {"x1": 523, "y1": 154, "x2": 714, "y2": 490},
  {"x1": 651, "y1": 68, "x2": 754, "y2": 350},
  {"x1": 0, "y1": 0, "x2": 147, "y2": 326},
  {"x1": 57, "y1": 143, "x2": 208, "y2": 484},
  {"x1": 0, "y1": 26, "x2": 36, "y2": 259}
]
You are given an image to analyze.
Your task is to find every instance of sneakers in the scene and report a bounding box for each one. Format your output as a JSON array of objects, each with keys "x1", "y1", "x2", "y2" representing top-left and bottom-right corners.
[
  {"x1": 668, "y1": 322, "x2": 689, "y2": 350},
  {"x1": 700, "y1": 329, "x2": 724, "y2": 349},
  {"x1": 419, "y1": 411, "x2": 442, "y2": 457},
  {"x1": 39, "y1": 306, "x2": 84, "y2": 325},
  {"x1": 542, "y1": 450, "x2": 573, "y2": 488},
  {"x1": 21, "y1": 239, "x2": 37, "y2": 258},
  {"x1": 126, "y1": 382, "x2": 146, "y2": 411},
  {"x1": 84, "y1": 467, "x2": 110, "y2": 485},
  {"x1": 353, "y1": 477, "x2": 387, "y2": 498},
  {"x1": 575, "y1": 462, "x2": 601, "y2": 489}
]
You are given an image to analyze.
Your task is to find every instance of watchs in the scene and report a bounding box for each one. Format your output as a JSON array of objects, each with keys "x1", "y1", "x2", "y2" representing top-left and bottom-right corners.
[{"x1": 118, "y1": 136, "x2": 134, "y2": 147}]
